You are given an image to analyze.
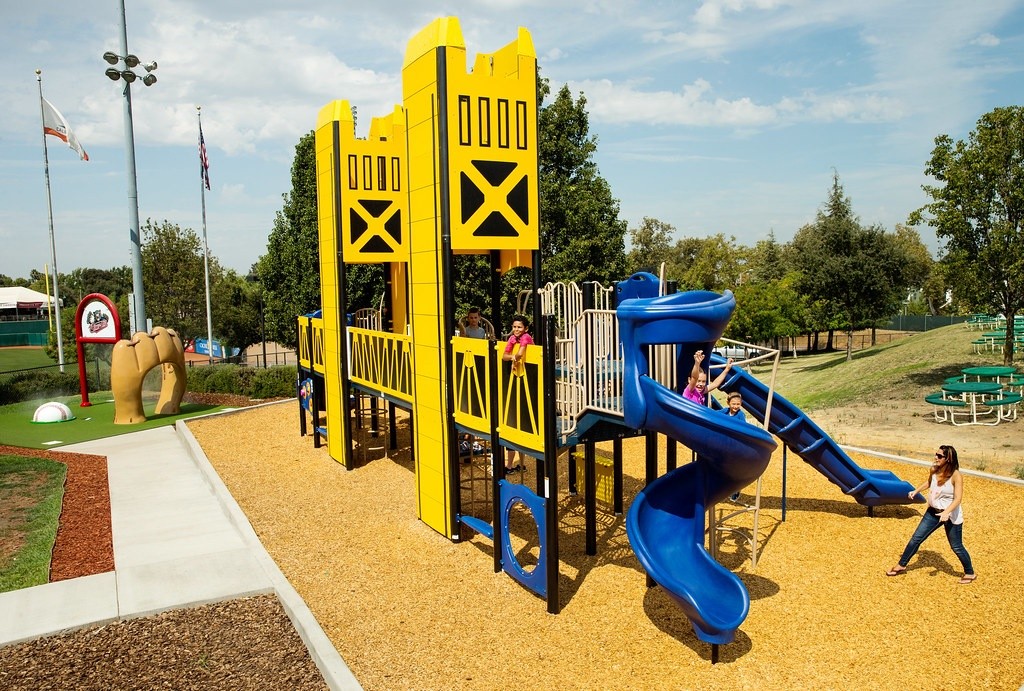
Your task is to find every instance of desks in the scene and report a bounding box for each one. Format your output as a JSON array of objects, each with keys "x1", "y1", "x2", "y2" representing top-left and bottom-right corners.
[
  {"x1": 1004, "y1": 322, "x2": 1024, "y2": 326},
  {"x1": 960, "y1": 366, "x2": 1017, "y2": 402},
  {"x1": 970, "y1": 313, "x2": 982, "y2": 320},
  {"x1": 976, "y1": 316, "x2": 990, "y2": 321},
  {"x1": 941, "y1": 381, "x2": 1004, "y2": 425},
  {"x1": 982, "y1": 332, "x2": 1019, "y2": 354},
  {"x1": 999, "y1": 325, "x2": 1024, "y2": 332}
]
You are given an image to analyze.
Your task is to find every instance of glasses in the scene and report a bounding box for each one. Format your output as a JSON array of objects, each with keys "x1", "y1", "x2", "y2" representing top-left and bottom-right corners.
[{"x1": 936, "y1": 453, "x2": 946, "y2": 459}]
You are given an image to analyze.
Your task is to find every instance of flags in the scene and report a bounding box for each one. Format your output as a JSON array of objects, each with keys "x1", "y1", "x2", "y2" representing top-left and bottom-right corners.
[
  {"x1": 198, "y1": 122, "x2": 211, "y2": 190},
  {"x1": 42, "y1": 95, "x2": 89, "y2": 161}
]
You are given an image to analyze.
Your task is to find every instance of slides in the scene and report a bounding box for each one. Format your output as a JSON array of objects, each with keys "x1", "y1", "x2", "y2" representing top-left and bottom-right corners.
[
  {"x1": 622, "y1": 373, "x2": 781, "y2": 645},
  {"x1": 709, "y1": 350, "x2": 928, "y2": 507}
]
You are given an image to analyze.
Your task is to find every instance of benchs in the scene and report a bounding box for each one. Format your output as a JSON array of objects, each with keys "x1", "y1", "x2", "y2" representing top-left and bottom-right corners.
[{"x1": 925, "y1": 320, "x2": 1024, "y2": 426}]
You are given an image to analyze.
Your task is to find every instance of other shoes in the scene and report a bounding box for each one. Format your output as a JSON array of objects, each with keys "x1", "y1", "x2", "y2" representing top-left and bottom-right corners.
[
  {"x1": 730, "y1": 491, "x2": 740, "y2": 502},
  {"x1": 515, "y1": 465, "x2": 527, "y2": 472},
  {"x1": 505, "y1": 467, "x2": 514, "y2": 474}
]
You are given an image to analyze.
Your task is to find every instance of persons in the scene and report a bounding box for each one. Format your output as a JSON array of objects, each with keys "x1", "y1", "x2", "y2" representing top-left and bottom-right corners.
[
  {"x1": 682, "y1": 350, "x2": 734, "y2": 405},
  {"x1": 465, "y1": 307, "x2": 486, "y2": 338},
  {"x1": 502, "y1": 316, "x2": 536, "y2": 371},
  {"x1": 716, "y1": 392, "x2": 747, "y2": 502},
  {"x1": 505, "y1": 447, "x2": 527, "y2": 474},
  {"x1": 886, "y1": 445, "x2": 977, "y2": 584}
]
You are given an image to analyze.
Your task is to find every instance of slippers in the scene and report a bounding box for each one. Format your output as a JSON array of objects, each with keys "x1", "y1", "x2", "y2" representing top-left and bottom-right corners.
[
  {"x1": 886, "y1": 567, "x2": 906, "y2": 576},
  {"x1": 958, "y1": 575, "x2": 977, "y2": 584}
]
